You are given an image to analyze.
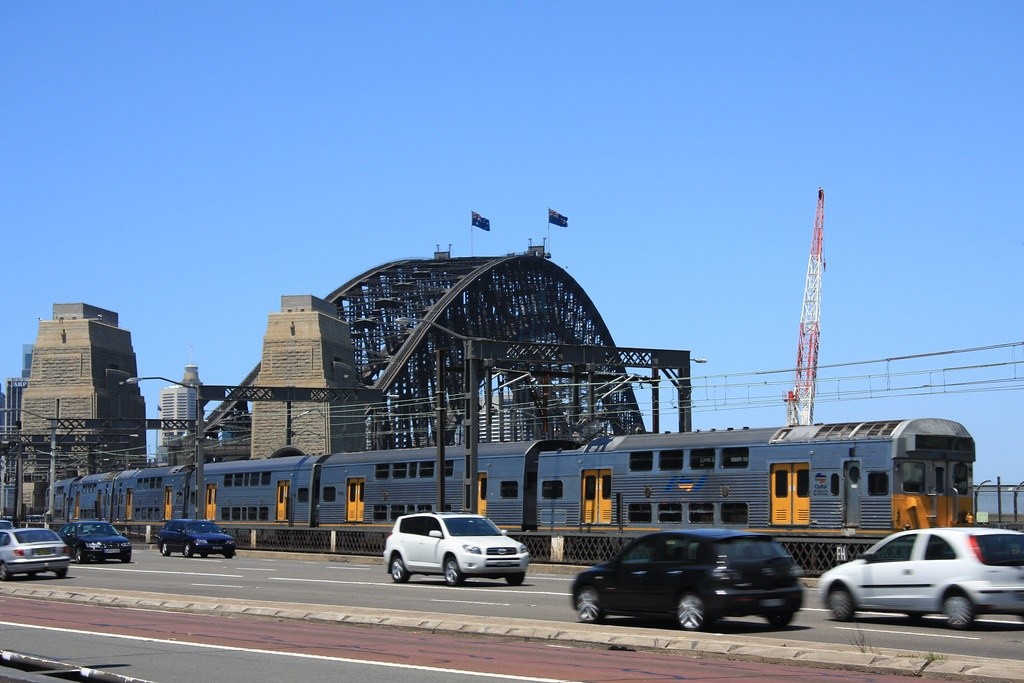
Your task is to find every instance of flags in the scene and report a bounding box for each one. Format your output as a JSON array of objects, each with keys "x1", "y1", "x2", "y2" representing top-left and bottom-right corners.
[
  {"x1": 471, "y1": 211, "x2": 490, "y2": 232},
  {"x1": 547, "y1": 208, "x2": 567, "y2": 228}
]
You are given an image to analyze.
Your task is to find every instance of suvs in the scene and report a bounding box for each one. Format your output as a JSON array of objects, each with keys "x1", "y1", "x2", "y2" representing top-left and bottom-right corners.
[{"x1": 382, "y1": 510, "x2": 530, "y2": 587}]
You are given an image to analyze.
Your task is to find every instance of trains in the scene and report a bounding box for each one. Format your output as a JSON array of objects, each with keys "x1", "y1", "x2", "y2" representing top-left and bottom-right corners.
[{"x1": 42, "y1": 415, "x2": 978, "y2": 554}]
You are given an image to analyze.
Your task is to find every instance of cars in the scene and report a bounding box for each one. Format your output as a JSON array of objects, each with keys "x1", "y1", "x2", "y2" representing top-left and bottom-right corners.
[
  {"x1": 0, "y1": 520, "x2": 15, "y2": 530},
  {"x1": 56, "y1": 520, "x2": 133, "y2": 565},
  {"x1": 156, "y1": 518, "x2": 235, "y2": 558},
  {"x1": 571, "y1": 529, "x2": 803, "y2": 633},
  {"x1": 0, "y1": 527, "x2": 70, "y2": 582},
  {"x1": 817, "y1": 527, "x2": 1024, "y2": 631}
]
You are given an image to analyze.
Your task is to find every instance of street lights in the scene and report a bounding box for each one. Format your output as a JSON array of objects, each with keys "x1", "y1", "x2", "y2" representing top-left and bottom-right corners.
[
  {"x1": 0, "y1": 406, "x2": 56, "y2": 520},
  {"x1": 125, "y1": 375, "x2": 206, "y2": 520},
  {"x1": 394, "y1": 316, "x2": 480, "y2": 515}
]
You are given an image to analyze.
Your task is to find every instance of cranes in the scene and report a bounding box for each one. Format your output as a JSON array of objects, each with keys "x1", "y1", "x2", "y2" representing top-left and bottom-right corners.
[{"x1": 785, "y1": 186, "x2": 828, "y2": 426}]
